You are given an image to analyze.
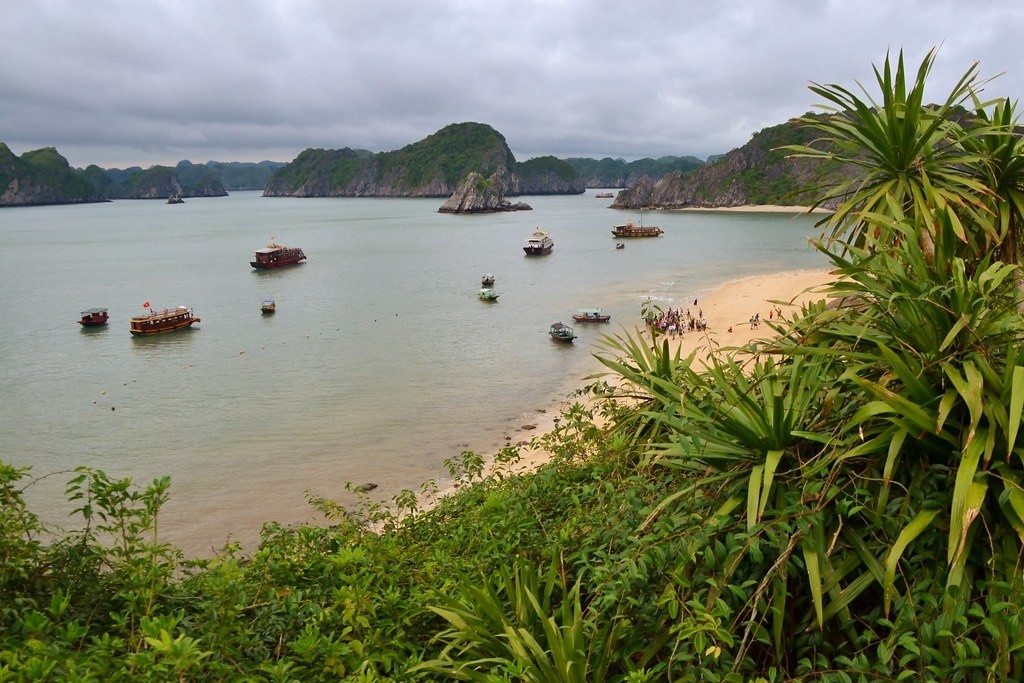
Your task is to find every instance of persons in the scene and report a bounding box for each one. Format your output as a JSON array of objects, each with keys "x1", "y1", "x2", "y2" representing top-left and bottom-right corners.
[{"x1": 645, "y1": 298, "x2": 782, "y2": 340}]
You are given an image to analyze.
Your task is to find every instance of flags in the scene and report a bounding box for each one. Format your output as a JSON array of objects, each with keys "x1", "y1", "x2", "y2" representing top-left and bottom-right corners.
[{"x1": 143, "y1": 302, "x2": 150, "y2": 307}]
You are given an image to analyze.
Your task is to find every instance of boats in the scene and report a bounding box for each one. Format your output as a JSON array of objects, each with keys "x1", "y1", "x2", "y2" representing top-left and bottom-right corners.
[
  {"x1": 250, "y1": 235, "x2": 307, "y2": 269},
  {"x1": 478, "y1": 289, "x2": 500, "y2": 301},
  {"x1": 77, "y1": 307, "x2": 110, "y2": 327},
  {"x1": 548, "y1": 321, "x2": 577, "y2": 342},
  {"x1": 130, "y1": 300, "x2": 200, "y2": 337},
  {"x1": 523, "y1": 225, "x2": 555, "y2": 255},
  {"x1": 260, "y1": 297, "x2": 276, "y2": 313},
  {"x1": 595, "y1": 191, "x2": 614, "y2": 198},
  {"x1": 572, "y1": 310, "x2": 611, "y2": 323},
  {"x1": 611, "y1": 208, "x2": 665, "y2": 237},
  {"x1": 482, "y1": 273, "x2": 496, "y2": 285}
]
